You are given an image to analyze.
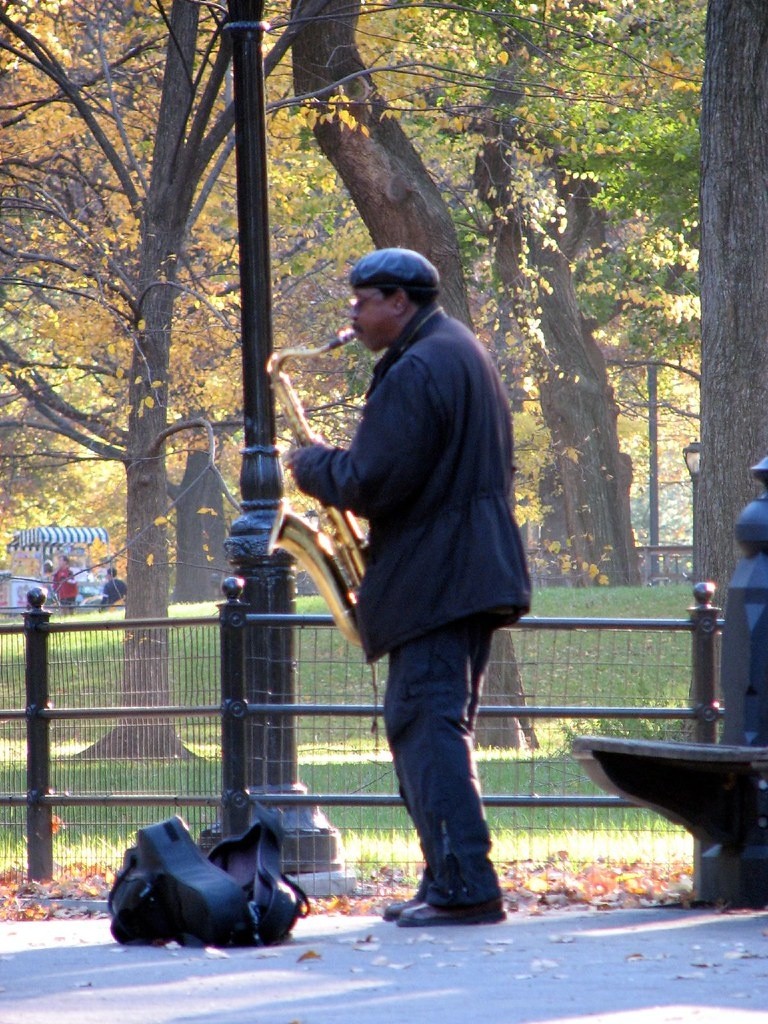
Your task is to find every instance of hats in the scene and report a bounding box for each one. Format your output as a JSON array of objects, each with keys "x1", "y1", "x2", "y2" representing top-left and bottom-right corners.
[{"x1": 349, "y1": 248, "x2": 439, "y2": 291}]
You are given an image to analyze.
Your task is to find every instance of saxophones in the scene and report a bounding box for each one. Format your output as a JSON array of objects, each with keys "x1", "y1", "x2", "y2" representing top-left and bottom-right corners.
[{"x1": 264, "y1": 326, "x2": 371, "y2": 648}]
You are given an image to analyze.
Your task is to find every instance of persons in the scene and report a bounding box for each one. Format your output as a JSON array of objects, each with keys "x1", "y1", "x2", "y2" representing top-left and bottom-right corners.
[
  {"x1": 284, "y1": 246, "x2": 531, "y2": 928},
  {"x1": 101, "y1": 567, "x2": 126, "y2": 610},
  {"x1": 52, "y1": 555, "x2": 78, "y2": 615}
]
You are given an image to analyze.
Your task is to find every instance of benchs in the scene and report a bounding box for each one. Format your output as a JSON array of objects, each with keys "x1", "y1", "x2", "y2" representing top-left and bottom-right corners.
[{"x1": 571, "y1": 733, "x2": 768, "y2": 910}]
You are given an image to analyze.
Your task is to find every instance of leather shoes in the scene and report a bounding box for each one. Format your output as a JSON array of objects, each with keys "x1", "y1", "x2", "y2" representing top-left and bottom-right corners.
[
  {"x1": 383, "y1": 899, "x2": 422, "y2": 921},
  {"x1": 397, "y1": 897, "x2": 507, "y2": 927}
]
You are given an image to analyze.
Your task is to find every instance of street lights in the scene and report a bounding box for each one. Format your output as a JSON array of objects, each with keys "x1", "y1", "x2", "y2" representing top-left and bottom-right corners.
[{"x1": 682, "y1": 438, "x2": 701, "y2": 580}]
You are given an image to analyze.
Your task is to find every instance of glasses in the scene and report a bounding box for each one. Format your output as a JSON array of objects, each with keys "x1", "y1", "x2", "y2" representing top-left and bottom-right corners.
[{"x1": 347, "y1": 289, "x2": 382, "y2": 314}]
